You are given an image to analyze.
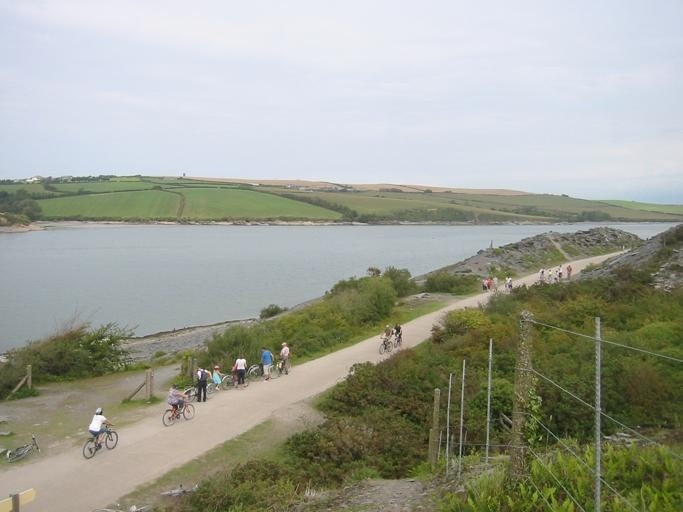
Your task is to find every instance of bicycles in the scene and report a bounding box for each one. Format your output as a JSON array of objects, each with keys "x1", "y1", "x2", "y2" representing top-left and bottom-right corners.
[
  {"x1": 182, "y1": 353, "x2": 292, "y2": 403},
  {"x1": 82, "y1": 423, "x2": 117, "y2": 459},
  {"x1": 160, "y1": 395, "x2": 195, "y2": 426},
  {"x1": 378, "y1": 333, "x2": 402, "y2": 354},
  {"x1": 7, "y1": 435, "x2": 40, "y2": 463}
]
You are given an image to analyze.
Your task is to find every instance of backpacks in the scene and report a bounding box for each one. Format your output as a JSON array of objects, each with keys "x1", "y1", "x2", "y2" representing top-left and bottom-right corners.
[{"x1": 199, "y1": 369, "x2": 208, "y2": 381}]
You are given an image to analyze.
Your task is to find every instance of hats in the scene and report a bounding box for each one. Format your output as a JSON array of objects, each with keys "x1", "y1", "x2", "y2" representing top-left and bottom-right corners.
[{"x1": 281, "y1": 342, "x2": 287, "y2": 345}]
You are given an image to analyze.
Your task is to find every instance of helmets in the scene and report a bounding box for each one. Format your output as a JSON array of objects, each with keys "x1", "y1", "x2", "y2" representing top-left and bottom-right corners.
[
  {"x1": 95, "y1": 407, "x2": 102, "y2": 415},
  {"x1": 214, "y1": 366, "x2": 220, "y2": 369}
]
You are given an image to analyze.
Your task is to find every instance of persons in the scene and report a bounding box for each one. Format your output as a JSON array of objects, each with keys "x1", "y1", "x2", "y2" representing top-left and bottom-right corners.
[
  {"x1": 381, "y1": 325, "x2": 392, "y2": 341},
  {"x1": 212, "y1": 365, "x2": 223, "y2": 391},
  {"x1": 88, "y1": 407, "x2": 112, "y2": 450},
  {"x1": 482, "y1": 275, "x2": 512, "y2": 293},
  {"x1": 231, "y1": 355, "x2": 248, "y2": 386},
  {"x1": 259, "y1": 347, "x2": 275, "y2": 380},
  {"x1": 539, "y1": 264, "x2": 572, "y2": 285},
  {"x1": 196, "y1": 366, "x2": 212, "y2": 402},
  {"x1": 168, "y1": 383, "x2": 188, "y2": 419},
  {"x1": 393, "y1": 323, "x2": 402, "y2": 340},
  {"x1": 279, "y1": 342, "x2": 289, "y2": 374}
]
